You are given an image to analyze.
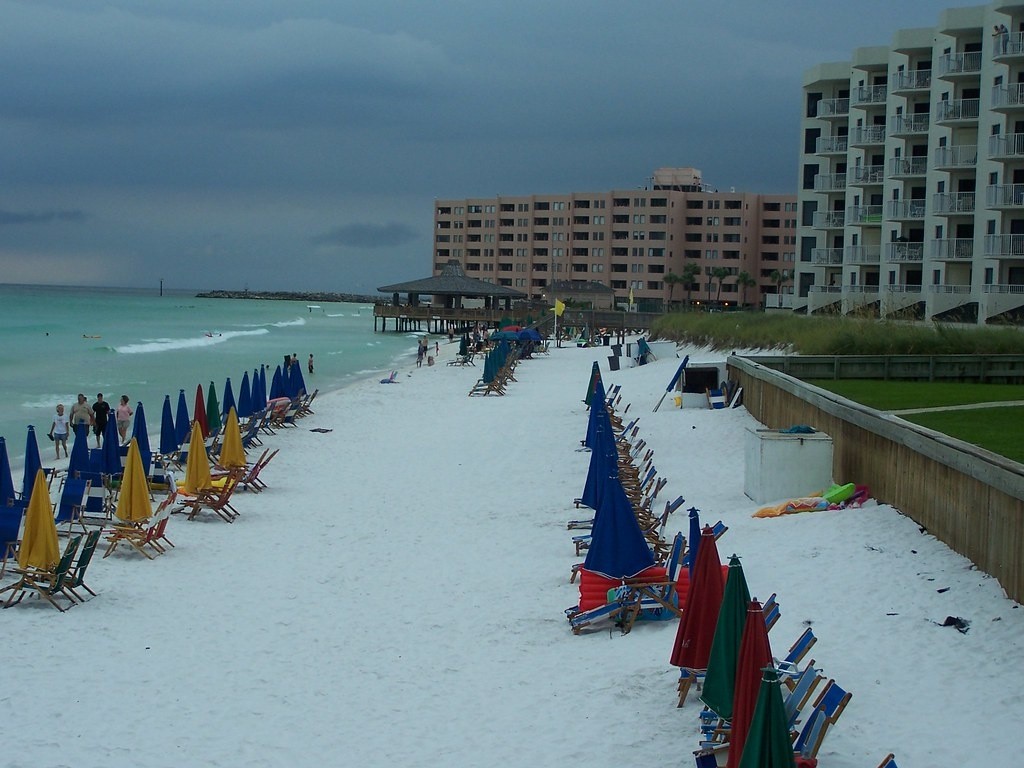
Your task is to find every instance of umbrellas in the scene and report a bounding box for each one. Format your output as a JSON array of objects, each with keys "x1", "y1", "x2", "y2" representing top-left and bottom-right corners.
[
  {"x1": 582, "y1": 361, "x2": 656, "y2": 630},
  {"x1": 1, "y1": 361, "x2": 97, "y2": 598},
  {"x1": 669, "y1": 508, "x2": 798, "y2": 768},
  {"x1": 97, "y1": 360, "x2": 307, "y2": 548},
  {"x1": 459, "y1": 326, "x2": 542, "y2": 396}
]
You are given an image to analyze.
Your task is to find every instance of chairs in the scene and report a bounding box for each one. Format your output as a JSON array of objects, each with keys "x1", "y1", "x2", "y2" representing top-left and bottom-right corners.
[
  {"x1": 565, "y1": 336, "x2": 897, "y2": 768},
  {"x1": 444, "y1": 330, "x2": 551, "y2": 396},
  {"x1": 0, "y1": 389, "x2": 321, "y2": 612},
  {"x1": 822, "y1": 56, "x2": 978, "y2": 294}
]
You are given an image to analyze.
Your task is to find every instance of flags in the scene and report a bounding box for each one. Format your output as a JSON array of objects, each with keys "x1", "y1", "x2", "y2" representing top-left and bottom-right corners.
[
  {"x1": 554, "y1": 299, "x2": 565, "y2": 316},
  {"x1": 629, "y1": 287, "x2": 634, "y2": 304}
]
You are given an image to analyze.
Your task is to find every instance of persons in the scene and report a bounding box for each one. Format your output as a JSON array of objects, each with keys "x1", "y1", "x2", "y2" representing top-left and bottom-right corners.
[
  {"x1": 117, "y1": 396, "x2": 133, "y2": 446},
  {"x1": 204, "y1": 332, "x2": 221, "y2": 338},
  {"x1": 473, "y1": 322, "x2": 489, "y2": 342},
  {"x1": 449, "y1": 326, "x2": 454, "y2": 343},
  {"x1": 416, "y1": 335, "x2": 428, "y2": 367},
  {"x1": 290, "y1": 353, "x2": 314, "y2": 374},
  {"x1": 92, "y1": 394, "x2": 109, "y2": 447},
  {"x1": 48, "y1": 404, "x2": 68, "y2": 460},
  {"x1": 69, "y1": 393, "x2": 95, "y2": 436},
  {"x1": 461, "y1": 303, "x2": 464, "y2": 309},
  {"x1": 375, "y1": 300, "x2": 431, "y2": 308},
  {"x1": 435, "y1": 342, "x2": 439, "y2": 356}
]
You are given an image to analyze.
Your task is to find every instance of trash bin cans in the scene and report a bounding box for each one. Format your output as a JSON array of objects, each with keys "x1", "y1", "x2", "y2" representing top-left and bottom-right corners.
[
  {"x1": 607, "y1": 355, "x2": 620, "y2": 370},
  {"x1": 603, "y1": 336, "x2": 610, "y2": 346},
  {"x1": 611, "y1": 344, "x2": 623, "y2": 356}
]
